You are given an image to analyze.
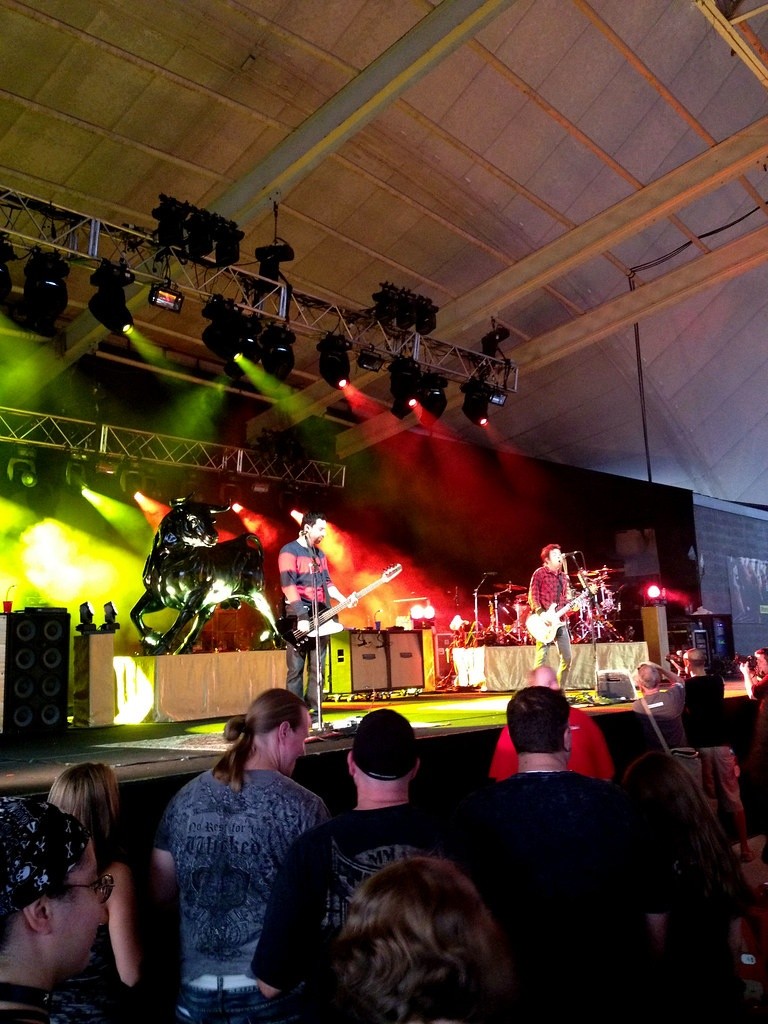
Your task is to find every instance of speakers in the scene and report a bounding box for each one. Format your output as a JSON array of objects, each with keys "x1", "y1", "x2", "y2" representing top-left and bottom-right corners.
[
  {"x1": 0, "y1": 612, "x2": 72, "y2": 736},
  {"x1": 391, "y1": 630, "x2": 425, "y2": 689},
  {"x1": 329, "y1": 630, "x2": 391, "y2": 694}
]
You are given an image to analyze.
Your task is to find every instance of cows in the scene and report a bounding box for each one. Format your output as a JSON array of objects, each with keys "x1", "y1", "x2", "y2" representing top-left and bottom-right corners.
[{"x1": 130, "y1": 490, "x2": 281, "y2": 654}]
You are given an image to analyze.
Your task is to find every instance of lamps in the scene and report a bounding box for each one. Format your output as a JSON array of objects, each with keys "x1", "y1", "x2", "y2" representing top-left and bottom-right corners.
[
  {"x1": 76, "y1": 601, "x2": 120, "y2": 636},
  {"x1": 642, "y1": 584, "x2": 667, "y2": 607},
  {"x1": 372, "y1": 282, "x2": 438, "y2": 335},
  {"x1": 7, "y1": 430, "x2": 329, "y2": 508},
  {"x1": 460, "y1": 378, "x2": 495, "y2": 429},
  {"x1": 0, "y1": 231, "x2": 16, "y2": 302},
  {"x1": 255, "y1": 242, "x2": 294, "y2": 293},
  {"x1": 151, "y1": 192, "x2": 244, "y2": 268},
  {"x1": 88, "y1": 260, "x2": 134, "y2": 335},
  {"x1": 200, "y1": 296, "x2": 297, "y2": 382},
  {"x1": 24, "y1": 247, "x2": 70, "y2": 315},
  {"x1": 387, "y1": 356, "x2": 449, "y2": 420},
  {"x1": 317, "y1": 332, "x2": 352, "y2": 390}
]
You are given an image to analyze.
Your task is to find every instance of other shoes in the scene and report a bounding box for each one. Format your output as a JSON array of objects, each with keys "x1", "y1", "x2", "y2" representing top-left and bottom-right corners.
[
  {"x1": 312, "y1": 721, "x2": 333, "y2": 730},
  {"x1": 741, "y1": 849, "x2": 756, "y2": 863}
]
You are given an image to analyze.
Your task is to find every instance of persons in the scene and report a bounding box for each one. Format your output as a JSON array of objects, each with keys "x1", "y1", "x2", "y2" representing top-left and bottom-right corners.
[
  {"x1": 0, "y1": 648, "x2": 768, "y2": 1024},
  {"x1": 278, "y1": 511, "x2": 358, "y2": 725},
  {"x1": 528, "y1": 543, "x2": 598, "y2": 689}
]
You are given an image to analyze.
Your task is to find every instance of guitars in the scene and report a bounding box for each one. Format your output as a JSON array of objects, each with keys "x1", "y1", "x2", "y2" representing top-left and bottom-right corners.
[
  {"x1": 525, "y1": 583, "x2": 598, "y2": 644},
  {"x1": 273, "y1": 563, "x2": 404, "y2": 654}
]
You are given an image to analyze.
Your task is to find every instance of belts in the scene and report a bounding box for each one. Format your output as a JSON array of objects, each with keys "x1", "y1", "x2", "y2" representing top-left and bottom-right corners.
[{"x1": 188, "y1": 974, "x2": 256, "y2": 990}]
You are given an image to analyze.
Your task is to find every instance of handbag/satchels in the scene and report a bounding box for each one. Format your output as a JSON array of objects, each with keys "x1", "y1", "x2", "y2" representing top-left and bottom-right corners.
[{"x1": 669, "y1": 747, "x2": 703, "y2": 790}]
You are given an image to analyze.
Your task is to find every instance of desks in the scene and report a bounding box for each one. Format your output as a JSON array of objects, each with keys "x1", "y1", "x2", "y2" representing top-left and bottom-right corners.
[{"x1": 452, "y1": 640, "x2": 649, "y2": 692}]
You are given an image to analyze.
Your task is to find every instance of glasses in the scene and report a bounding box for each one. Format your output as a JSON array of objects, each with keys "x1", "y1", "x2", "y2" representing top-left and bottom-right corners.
[{"x1": 65, "y1": 874, "x2": 114, "y2": 903}]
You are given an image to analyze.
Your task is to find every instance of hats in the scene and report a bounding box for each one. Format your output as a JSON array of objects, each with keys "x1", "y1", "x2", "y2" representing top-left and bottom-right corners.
[
  {"x1": 351, "y1": 708, "x2": 417, "y2": 781},
  {"x1": 1, "y1": 795, "x2": 93, "y2": 918}
]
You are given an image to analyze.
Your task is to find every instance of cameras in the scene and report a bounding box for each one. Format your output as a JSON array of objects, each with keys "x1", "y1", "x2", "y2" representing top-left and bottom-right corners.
[
  {"x1": 733, "y1": 652, "x2": 758, "y2": 669},
  {"x1": 665, "y1": 654, "x2": 685, "y2": 668}
]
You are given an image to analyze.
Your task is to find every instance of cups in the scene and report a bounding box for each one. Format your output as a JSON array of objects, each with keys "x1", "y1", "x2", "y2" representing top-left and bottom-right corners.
[
  {"x1": 375, "y1": 621, "x2": 380, "y2": 629},
  {"x1": 3, "y1": 601, "x2": 13, "y2": 613}
]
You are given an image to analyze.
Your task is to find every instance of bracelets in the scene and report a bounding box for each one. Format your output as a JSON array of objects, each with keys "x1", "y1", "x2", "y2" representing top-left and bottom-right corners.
[{"x1": 750, "y1": 674, "x2": 754, "y2": 679}]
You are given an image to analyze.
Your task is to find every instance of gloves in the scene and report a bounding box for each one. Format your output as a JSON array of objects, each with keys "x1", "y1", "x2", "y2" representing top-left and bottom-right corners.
[{"x1": 296, "y1": 612, "x2": 310, "y2": 632}]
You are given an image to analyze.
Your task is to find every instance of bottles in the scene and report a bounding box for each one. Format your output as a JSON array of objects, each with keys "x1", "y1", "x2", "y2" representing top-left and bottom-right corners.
[
  {"x1": 459, "y1": 626, "x2": 465, "y2": 647},
  {"x1": 698, "y1": 619, "x2": 703, "y2": 630}
]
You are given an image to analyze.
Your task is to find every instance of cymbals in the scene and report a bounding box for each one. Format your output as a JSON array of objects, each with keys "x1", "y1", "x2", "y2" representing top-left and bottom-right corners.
[
  {"x1": 473, "y1": 594, "x2": 494, "y2": 599},
  {"x1": 493, "y1": 583, "x2": 527, "y2": 591},
  {"x1": 569, "y1": 570, "x2": 599, "y2": 576},
  {"x1": 595, "y1": 568, "x2": 621, "y2": 573}
]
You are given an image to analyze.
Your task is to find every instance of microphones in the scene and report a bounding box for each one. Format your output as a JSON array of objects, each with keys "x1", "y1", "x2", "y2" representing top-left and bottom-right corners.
[
  {"x1": 298, "y1": 530, "x2": 308, "y2": 536},
  {"x1": 484, "y1": 572, "x2": 498, "y2": 576},
  {"x1": 560, "y1": 550, "x2": 578, "y2": 559}
]
